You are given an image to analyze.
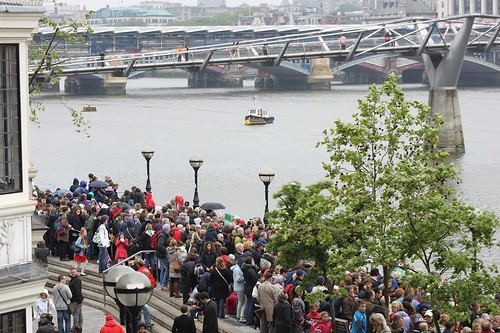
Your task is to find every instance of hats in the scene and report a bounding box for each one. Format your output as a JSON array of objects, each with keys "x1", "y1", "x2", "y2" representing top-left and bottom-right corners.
[
  {"x1": 101, "y1": 215, "x2": 110, "y2": 220},
  {"x1": 105, "y1": 314, "x2": 116, "y2": 322},
  {"x1": 423, "y1": 309, "x2": 434, "y2": 316}
]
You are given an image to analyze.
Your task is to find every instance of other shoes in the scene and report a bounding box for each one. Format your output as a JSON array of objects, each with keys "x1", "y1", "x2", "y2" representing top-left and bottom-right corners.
[
  {"x1": 49, "y1": 252, "x2": 116, "y2": 276},
  {"x1": 150, "y1": 272, "x2": 260, "y2": 333}
]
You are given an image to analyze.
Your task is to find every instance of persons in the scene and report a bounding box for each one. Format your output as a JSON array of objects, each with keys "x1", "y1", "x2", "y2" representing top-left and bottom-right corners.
[
  {"x1": 53, "y1": 274, "x2": 73, "y2": 332},
  {"x1": 32, "y1": 171, "x2": 292, "y2": 272},
  {"x1": 137, "y1": 303, "x2": 154, "y2": 327},
  {"x1": 241, "y1": 256, "x2": 257, "y2": 325},
  {"x1": 38, "y1": 313, "x2": 54, "y2": 327},
  {"x1": 45, "y1": 53, "x2": 52, "y2": 70},
  {"x1": 230, "y1": 258, "x2": 247, "y2": 321},
  {"x1": 184, "y1": 44, "x2": 189, "y2": 60},
  {"x1": 100, "y1": 49, "x2": 105, "y2": 66},
  {"x1": 293, "y1": 261, "x2": 500, "y2": 332},
  {"x1": 272, "y1": 291, "x2": 294, "y2": 332},
  {"x1": 177, "y1": 45, "x2": 182, "y2": 61},
  {"x1": 178, "y1": 254, "x2": 197, "y2": 304},
  {"x1": 73, "y1": 325, "x2": 82, "y2": 333},
  {"x1": 190, "y1": 293, "x2": 204, "y2": 318},
  {"x1": 171, "y1": 305, "x2": 197, "y2": 333},
  {"x1": 284, "y1": 275, "x2": 293, "y2": 295},
  {"x1": 257, "y1": 272, "x2": 278, "y2": 332},
  {"x1": 200, "y1": 291, "x2": 218, "y2": 332},
  {"x1": 385, "y1": 31, "x2": 390, "y2": 46},
  {"x1": 208, "y1": 257, "x2": 227, "y2": 318},
  {"x1": 262, "y1": 39, "x2": 268, "y2": 54},
  {"x1": 155, "y1": 222, "x2": 169, "y2": 290},
  {"x1": 33, "y1": 288, "x2": 54, "y2": 320},
  {"x1": 68, "y1": 266, "x2": 84, "y2": 331},
  {"x1": 391, "y1": 31, "x2": 396, "y2": 46},
  {"x1": 100, "y1": 314, "x2": 125, "y2": 333},
  {"x1": 340, "y1": 34, "x2": 347, "y2": 49},
  {"x1": 166, "y1": 238, "x2": 182, "y2": 298},
  {"x1": 137, "y1": 323, "x2": 148, "y2": 333},
  {"x1": 231, "y1": 41, "x2": 236, "y2": 56},
  {"x1": 251, "y1": 273, "x2": 264, "y2": 329},
  {"x1": 276, "y1": 267, "x2": 287, "y2": 284},
  {"x1": 233, "y1": 40, "x2": 240, "y2": 57}
]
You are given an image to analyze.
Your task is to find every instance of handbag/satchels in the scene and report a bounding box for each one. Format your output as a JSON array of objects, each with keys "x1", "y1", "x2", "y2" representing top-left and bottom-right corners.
[
  {"x1": 67, "y1": 301, "x2": 78, "y2": 315},
  {"x1": 69, "y1": 243, "x2": 84, "y2": 253}
]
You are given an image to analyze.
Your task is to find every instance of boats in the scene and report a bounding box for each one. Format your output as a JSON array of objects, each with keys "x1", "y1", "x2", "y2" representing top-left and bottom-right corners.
[{"x1": 245, "y1": 108, "x2": 275, "y2": 127}]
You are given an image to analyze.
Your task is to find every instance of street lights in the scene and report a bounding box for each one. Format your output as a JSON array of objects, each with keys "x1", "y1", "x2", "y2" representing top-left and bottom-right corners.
[
  {"x1": 141, "y1": 146, "x2": 155, "y2": 195},
  {"x1": 259, "y1": 167, "x2": 276, "y2": 230},
  {"x1": 188, "y1": 154, "x2": 204, "y2": 210}
]
